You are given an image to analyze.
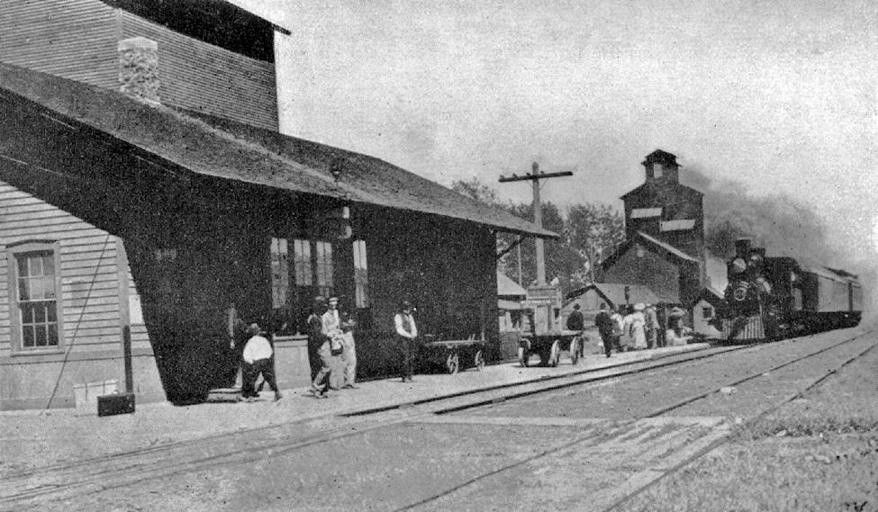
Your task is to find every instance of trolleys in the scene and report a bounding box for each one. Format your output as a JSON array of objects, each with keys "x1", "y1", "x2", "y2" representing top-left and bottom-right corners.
[
  {"x1": 518, "y1": 329, "x2": 582, "y2": 366},
  {"x1": 421, "y1": 339, "x2": 491, "y2": 374}
]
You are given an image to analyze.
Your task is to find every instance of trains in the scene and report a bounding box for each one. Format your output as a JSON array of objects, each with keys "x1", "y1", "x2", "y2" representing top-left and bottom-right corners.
[{"x1": 708, "y1": 238, "x2": 863, "y2": 344}]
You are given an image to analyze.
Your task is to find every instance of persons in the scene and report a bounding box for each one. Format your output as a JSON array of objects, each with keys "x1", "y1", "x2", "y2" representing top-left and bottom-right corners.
[
  {"x1": 306, "y1": 293, "x2": 358, "y2": 399},
  {"x1": 394, "y1": 301, "x2": 419, "y2": 383},
  {"x1": 567, "y1": 303, "x2": 584, "y2": 357},
  {"x1": 668, "y1": 306, "x2": 687, "y2": 338},
  {"x1": 594, "y1": 301, "x2": 667, "y2": 357},
  {"x1": 237, "y1": 302, "x2": 284, "y2": 402}
]
